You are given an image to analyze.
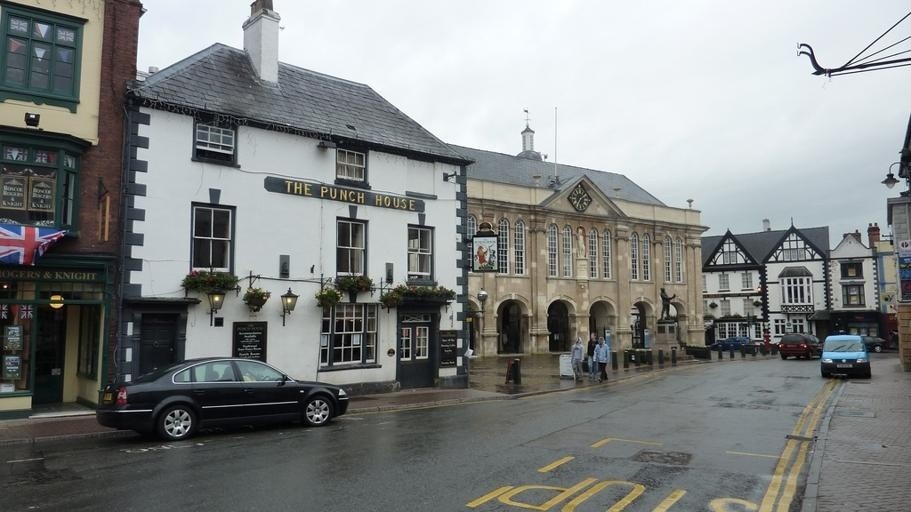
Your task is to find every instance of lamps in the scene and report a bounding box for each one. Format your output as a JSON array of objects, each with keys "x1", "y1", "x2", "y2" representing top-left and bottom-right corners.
[
  {"x1": 206, "y1": 289, "x2": 225, "y2": 325},
  {"x1": 280, "y1": 288, "x2": 298, "y2": 325}
]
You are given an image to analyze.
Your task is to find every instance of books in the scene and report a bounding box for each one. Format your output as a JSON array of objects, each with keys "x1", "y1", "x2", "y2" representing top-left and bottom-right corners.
[
  {"x1": 5, "y1": 325, "x2": 25, "y2": 349},
  {"x1": 3, "y1": 354, "x2": 22, "y2": 379}
]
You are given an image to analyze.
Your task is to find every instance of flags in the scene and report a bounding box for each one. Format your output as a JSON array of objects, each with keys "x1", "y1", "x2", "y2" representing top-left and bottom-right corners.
[
  {"x1": 19, "y1": 304, "x2": 35, "y2": 319},
  {"x1": 1, "y1": 304, "x2": 8, "y2": 320},
  {"x1": 0, "y1": 223, "x2": 70, "y2": 268}
]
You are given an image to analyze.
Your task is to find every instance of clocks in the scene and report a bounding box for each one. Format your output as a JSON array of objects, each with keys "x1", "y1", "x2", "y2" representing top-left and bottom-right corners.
[{"x1": 568, "y1": 183, "x2": 593, "y2": 213}]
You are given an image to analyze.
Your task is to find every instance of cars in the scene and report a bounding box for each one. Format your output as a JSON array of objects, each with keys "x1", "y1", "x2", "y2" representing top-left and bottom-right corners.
[
  {"x1": 95, "y1": 356, "x2": 350, "y2": 440},
  {"x1": 778, "y1": 334, "x2": 890, "y2": 378},
  {"x1": 711, "y1": 336, "x2": 750, "y2": 351}
]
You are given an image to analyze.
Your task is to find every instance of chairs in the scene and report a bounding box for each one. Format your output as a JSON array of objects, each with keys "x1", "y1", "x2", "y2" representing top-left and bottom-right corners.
[
  {"x1": 221, "y1": 367, "x2": 236, "y2": 381},
  {"x1": 207, "y1": 372, "x2": 219, "y2": 381}
]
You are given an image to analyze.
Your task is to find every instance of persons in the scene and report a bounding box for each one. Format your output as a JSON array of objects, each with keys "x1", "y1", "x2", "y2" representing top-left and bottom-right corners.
[
  {"x1": 588, "y1": 332, "x2": 600, "y2": 381},
  {"x1": 571, "y1": 336, "x2": 587, "y2": 381},
  {"x1": 594, "y1": 336, "x2": 611, "y2": 381},
  {"x1": 660, "y1": 286, "x2": 676, "y2": 320}
]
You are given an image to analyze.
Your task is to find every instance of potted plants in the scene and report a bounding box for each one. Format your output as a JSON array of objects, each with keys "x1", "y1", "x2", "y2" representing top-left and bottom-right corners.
[{"x1": 243, "y1": 287, "x2": 271, "y2": 308}]
